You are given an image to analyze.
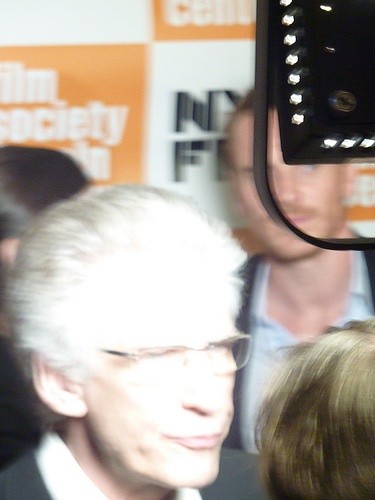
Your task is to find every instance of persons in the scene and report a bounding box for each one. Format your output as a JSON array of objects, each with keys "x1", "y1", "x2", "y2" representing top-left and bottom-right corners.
[
  {"x1": 0, "y1": 179, "x2": 270, "y2": 500},
  {"x1": 250, "y1": 316, "x2": 375, "y2": 500},
  {"x1": 0, "y1": 144, "x2": 93, "y2": 473},
  {"x1": 220, "y1": 88, "x2": 375, "y2": 455}
]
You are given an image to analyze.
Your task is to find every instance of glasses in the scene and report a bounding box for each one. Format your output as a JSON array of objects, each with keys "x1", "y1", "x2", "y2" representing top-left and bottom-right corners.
[{"x1": 103, "y1": 329, "x2": 254, "y2": 374}]
